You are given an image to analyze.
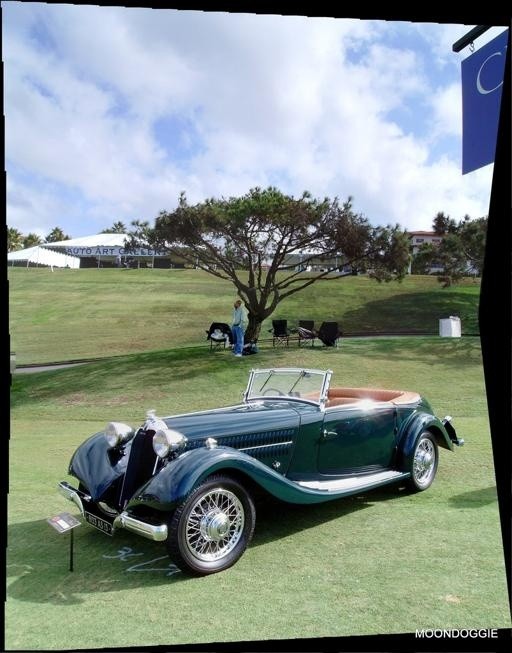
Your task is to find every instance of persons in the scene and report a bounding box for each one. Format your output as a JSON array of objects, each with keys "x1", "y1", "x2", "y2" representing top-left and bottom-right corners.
[
  {"x1": 230, "y1": 300, "x2": 245, "y2": 357},
  {"x1": 115, "y1": 254, "x2": 121, "y2": 268}
]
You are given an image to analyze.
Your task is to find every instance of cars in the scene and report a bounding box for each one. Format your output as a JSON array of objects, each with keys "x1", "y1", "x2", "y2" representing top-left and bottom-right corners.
[
  {"x1": 59, "y1": 365, "x2": 464, "y2": 579},
  {"x1": 463, "y1": 269, "x2": 480, "y2": 277}
]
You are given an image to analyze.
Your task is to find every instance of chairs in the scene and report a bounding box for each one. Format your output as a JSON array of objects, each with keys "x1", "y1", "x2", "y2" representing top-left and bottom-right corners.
[
  {"x1": 319, "y1": 322, "x2": 341, "y2": 348},
  {"x1": 298, "y1": 320, "x2": 314, "y2": 347},
  {"x1": 206, "y1": 322, "x2": 227, "y2": 349},
  {"x1": 269, "y1": 320, "x2": 289, "y2": 348}
]
